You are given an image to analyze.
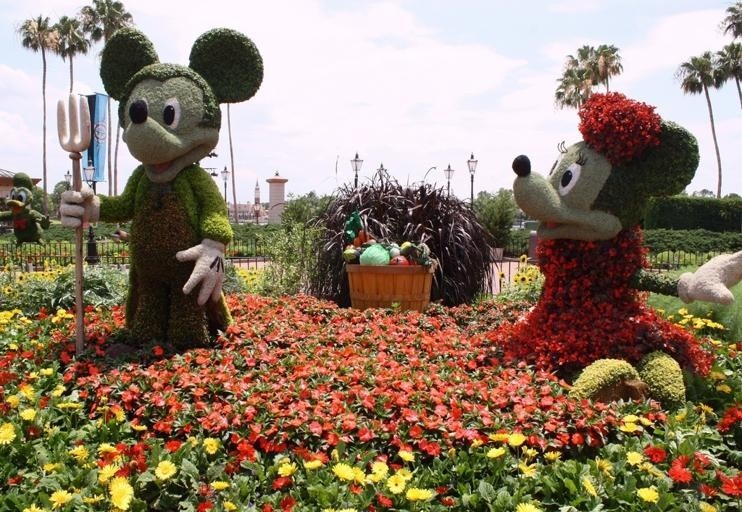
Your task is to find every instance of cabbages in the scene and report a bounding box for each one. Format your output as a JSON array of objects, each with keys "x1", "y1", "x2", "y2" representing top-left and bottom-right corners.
[{"x1": 360, "y1": 243, "x2": 391, "y2": 266}]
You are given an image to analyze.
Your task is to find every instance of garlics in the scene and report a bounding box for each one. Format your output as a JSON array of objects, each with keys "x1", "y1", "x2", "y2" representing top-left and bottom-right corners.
[{"x1": 390, "y1": 247, "x2": 400, "y2": 258}]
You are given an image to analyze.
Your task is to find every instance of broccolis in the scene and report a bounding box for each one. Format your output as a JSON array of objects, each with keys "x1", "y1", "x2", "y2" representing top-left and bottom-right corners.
[{"x1": 400, "y1": 242, "x2": 431, "y2": 265}]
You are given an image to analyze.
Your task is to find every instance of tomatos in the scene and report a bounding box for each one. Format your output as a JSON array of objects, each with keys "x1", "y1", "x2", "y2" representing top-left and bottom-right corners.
[{"x1": 390, "y1": 255, "x2": 410, "y2": 266}]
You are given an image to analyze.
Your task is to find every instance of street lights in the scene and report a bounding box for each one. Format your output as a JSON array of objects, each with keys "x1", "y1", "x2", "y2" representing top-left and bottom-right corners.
[
  {"x1": 252, "y1": 201, "x2": 262, "y2": 225},
  {"x1": 195, "y1": 161, "x2": 218, "y2": 177},
  {"x1": 377, "y1": 163, "x2": 387, "y2": 190},
  {"x1": 466, "y1": 153, "x2": 478, "y2": 212},
  {"x1": 221, "y1": 166, "x2": 230, "y2": 204},
  {"x1": 83, "y1": 160, "x2": 101, "y2": 266},
  {"x1": 350, "y1": 152, "x2": 364, "y2": 188},
  {"x1": 443, "y1": 164, "x2": 455, "y2": 199},
  {"x1": 519, "y1": 207, "x2": 524, "y2": 229},
  {"x1": 65, "y1": 170, "x2": 73, "y2": 191}
]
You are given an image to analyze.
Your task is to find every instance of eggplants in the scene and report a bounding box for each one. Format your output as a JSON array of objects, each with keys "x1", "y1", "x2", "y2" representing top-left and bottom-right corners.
[{"x1": 343, "y1": 248, "x2": 361, "y2": 264}]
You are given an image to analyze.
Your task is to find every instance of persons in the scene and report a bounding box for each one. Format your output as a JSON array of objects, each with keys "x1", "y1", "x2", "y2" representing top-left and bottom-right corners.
[{"x1": 111, "y1": 229, "x2": 132, "y2": 245}]
